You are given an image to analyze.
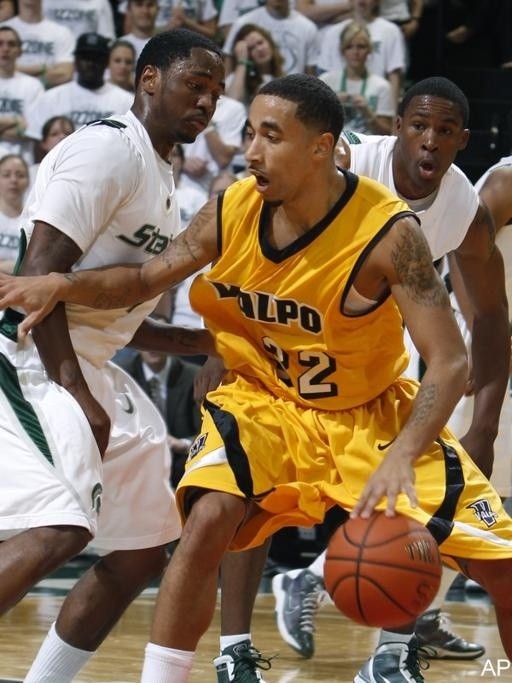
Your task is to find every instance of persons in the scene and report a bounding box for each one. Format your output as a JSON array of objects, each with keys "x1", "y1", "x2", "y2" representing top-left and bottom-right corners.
[
  {"x1": 0, "y1": 29, "x2": 220, "y2": 683},
  {"x1": 193, "y1": 76, "x2": 512, "y2": 683},
  {"x1": 0, "y1": 0, "x2": 512, "y2": 600},
  {"x1": 271, "y1": 154, "x2": 512, "y2": 661},
  {"x1": 0, "y1": 73, "x2": 512, "y2": 683}
]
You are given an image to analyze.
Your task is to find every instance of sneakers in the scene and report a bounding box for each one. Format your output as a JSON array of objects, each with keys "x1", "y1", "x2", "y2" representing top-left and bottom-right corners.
[
  {"x1": 271, "y1": 567, "x2": 328, "y2": 659},
  {"x1": 351, "y1": 640, "x2": 430, "y2": 683},
  {"x1": 412, "y1": 608, "x2": 486, "y2": 660},
  {"x1": 213, "y1": 638, "x2": 279, "y2": 682}
]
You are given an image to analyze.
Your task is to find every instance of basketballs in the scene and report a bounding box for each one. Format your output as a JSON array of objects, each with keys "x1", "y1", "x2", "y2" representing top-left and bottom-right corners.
[{"x1": 323, "y1": 509, "x2": 441, "y2": 628}]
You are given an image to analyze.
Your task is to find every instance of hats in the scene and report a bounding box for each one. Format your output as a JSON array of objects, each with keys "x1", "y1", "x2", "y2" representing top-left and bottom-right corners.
[{"x1": 70, "y1": 30, "x2": 110, "y2": 57}]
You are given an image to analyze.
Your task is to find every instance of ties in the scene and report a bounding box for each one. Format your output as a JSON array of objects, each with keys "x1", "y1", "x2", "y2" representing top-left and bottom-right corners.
[{"x1": 147, "y1": 376, "x2": 163, "y2": 419}]
